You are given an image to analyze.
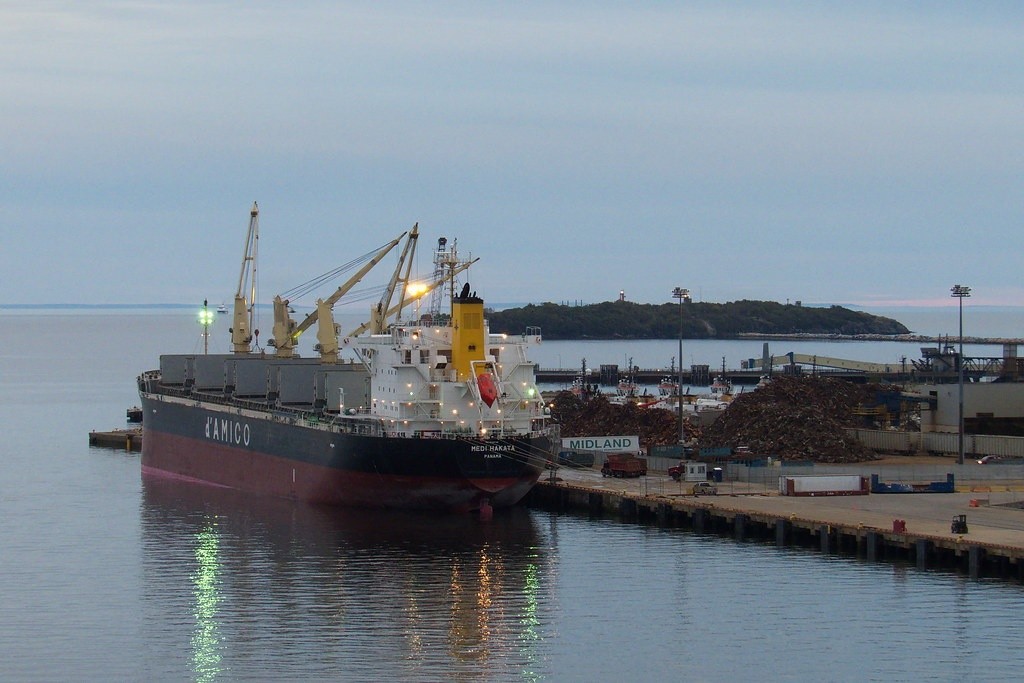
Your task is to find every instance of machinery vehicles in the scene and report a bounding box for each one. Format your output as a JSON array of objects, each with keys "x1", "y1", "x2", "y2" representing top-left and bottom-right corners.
[{"x1": 668, "y1": 461, "x2": 687, "y2": 480}]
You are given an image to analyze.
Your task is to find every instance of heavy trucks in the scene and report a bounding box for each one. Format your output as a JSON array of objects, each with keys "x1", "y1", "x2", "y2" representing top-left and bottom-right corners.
[
  {"x1": 558, "y1": 451, "x2": 594, "y2": 468},
  {"x1": 601, "y1": 455, "x2": 647, "y2": 479}
]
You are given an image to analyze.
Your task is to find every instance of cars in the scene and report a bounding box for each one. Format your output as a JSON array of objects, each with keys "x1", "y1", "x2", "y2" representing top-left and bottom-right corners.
[{"x1": 978, "y1": 455, "x2": 1002, "y2": 464}]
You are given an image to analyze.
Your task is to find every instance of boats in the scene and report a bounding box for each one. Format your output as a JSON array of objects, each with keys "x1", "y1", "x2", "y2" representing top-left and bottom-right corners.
[
  {"x1": 657, "y1": 378, "x2": 680, "y2": 396},
  {"x1": 710, "y1": 375, "x2": 733, "y2": 394},
  {"x1": 143, "y1": 199, "x2": 561, "y2": 536},
  {"x1": 615, "y1": 377, "x2": 639, "y2": 395},
  {"x1": 216, "y1": 303, "x2": 229, "y2": 314},
  {"x1": 569, "y1": 377, "x2": 601, "y2": 395}
]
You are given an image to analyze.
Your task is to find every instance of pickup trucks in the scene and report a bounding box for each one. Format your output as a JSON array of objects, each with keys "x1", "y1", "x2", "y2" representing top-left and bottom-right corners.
[{"x1": 693, "y1": 482, "x2": 718, "y2": 496}]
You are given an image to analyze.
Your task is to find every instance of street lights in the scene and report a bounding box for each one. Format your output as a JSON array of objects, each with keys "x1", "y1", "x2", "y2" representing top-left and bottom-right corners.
[
  {"x1": 672, "y1": 286, "x2": 691, "y2": 443},
  {"x1": 197, "y1": 310, "x2": 213, "y2": 355},
  {"x1": 951, "y1": 284, "x2": 971, "y2": 465},
  {"x1": 408, "y1": 283, "x2": 427, "y2": 325}
]
